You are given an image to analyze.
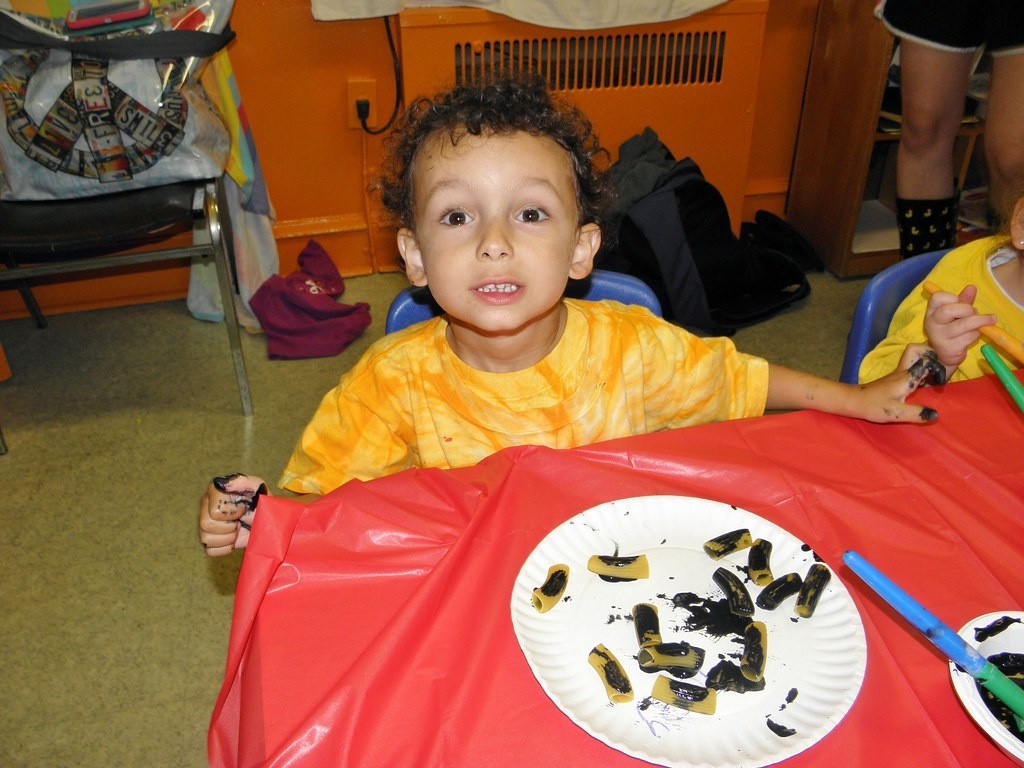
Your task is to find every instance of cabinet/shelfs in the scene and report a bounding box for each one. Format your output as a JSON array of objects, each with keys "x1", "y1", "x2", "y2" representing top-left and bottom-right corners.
[{"x1": 787, "y1": 0, "x2": 1003, "y2": 282}]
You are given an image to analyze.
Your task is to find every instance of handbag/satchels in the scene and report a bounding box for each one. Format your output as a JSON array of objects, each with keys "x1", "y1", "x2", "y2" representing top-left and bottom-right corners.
[{"x1": 0, "y1": 0, "x2": 237, "y2": 202}]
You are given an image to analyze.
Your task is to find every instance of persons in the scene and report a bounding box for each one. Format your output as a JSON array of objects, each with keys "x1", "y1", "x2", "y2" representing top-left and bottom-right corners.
[
  {"x1": 858, "y1": 192, "x2": 1024, "y2": 388},
  {"x1": 880, "y1": 0, "x2": 1024, "y2": 259},
  {"x1": 198, "y1": 65, "x2": 947, "y2": 559}
]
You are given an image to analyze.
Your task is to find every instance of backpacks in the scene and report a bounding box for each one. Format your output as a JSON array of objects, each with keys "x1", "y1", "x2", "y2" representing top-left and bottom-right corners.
[{"x1": 595, "y1": 156, "x2": 812, "y2": 338}]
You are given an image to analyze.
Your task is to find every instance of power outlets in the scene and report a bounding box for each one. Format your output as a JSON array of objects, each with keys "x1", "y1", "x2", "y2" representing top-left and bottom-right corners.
[{"x1": 346, "y1": 77, "x2": 378, "y2": 130}]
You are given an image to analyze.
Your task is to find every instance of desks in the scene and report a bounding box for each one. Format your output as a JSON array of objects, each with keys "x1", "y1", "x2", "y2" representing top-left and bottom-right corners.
[{"x1": 207, "y1": 369, "x2": 1024, "y2": 768}]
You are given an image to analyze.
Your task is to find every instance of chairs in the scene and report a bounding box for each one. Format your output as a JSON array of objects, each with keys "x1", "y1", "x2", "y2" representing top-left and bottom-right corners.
[
  {"x1": 838, "y1": 247, "x2": 962, "y2": 385},
  {"x1": 0, "y1": 0, "x2": 254, "y2": 457},
  {"x1": 383, "y1": 267, "x2": 663, "y2": 336}
]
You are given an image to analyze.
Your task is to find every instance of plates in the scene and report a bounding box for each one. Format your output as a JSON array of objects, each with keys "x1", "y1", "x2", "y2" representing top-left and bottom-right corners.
[
  {"x1": 949, "y1": 611, "x2": 1024, "y2": 767},
  {"x1": 510, "y1": 494, "x2": 866, "y2": 768}
]
